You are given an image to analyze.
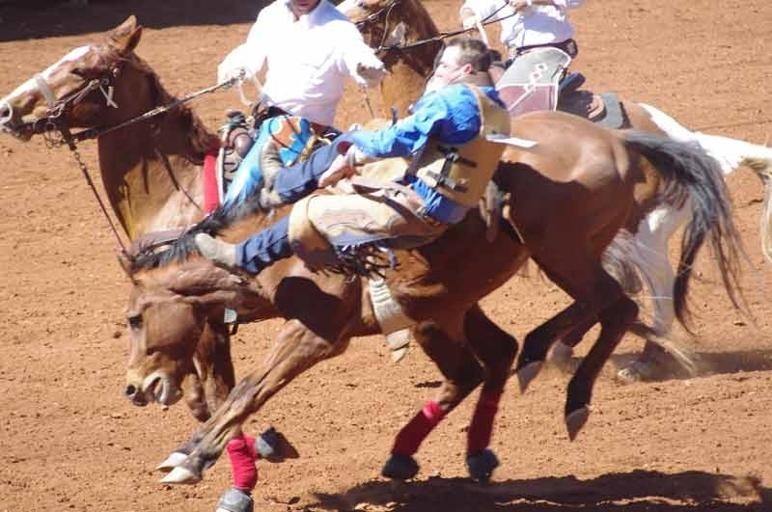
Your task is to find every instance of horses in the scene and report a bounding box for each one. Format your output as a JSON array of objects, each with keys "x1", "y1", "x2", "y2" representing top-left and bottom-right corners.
[
  {"x1": 291, "y1": 190, "x2": 300, "y2": 215},
  {"x1": 0, "y1": 1, "x2": 771, "y2": 512}
]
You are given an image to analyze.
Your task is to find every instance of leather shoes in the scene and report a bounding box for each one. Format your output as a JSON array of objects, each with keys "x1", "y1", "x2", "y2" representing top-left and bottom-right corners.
[
  {"x1": 194, "y1": 233, "x2": 254, "y2": 286},
  {"x1": 258, "y1": 142, "x2": 287, "y2": 211}
]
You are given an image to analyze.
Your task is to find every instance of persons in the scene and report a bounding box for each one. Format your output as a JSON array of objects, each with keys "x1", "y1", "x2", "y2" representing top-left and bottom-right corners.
[
  {"x1": 193, "y1": 38, "x2": 510, "y2": 286},
  {"x1": 217, "y1": 0, "x2": 388, "y2": 334},
  {"x1": 460, "y1": 0, "x2": 577, "y2": 118}
]
